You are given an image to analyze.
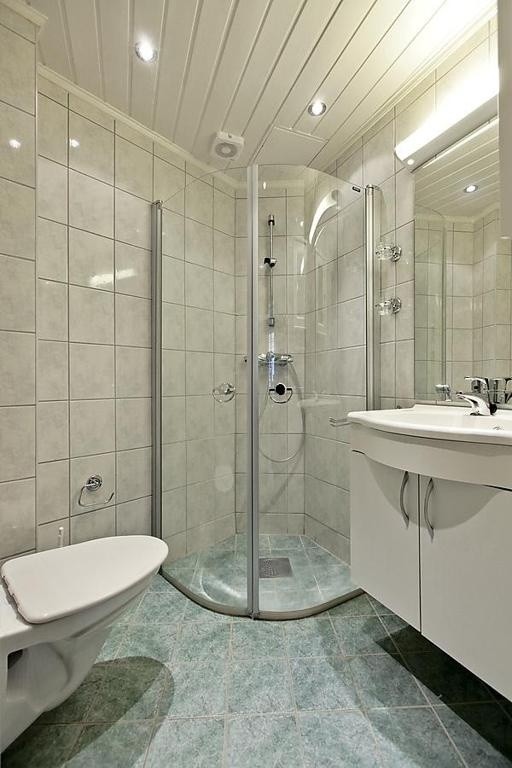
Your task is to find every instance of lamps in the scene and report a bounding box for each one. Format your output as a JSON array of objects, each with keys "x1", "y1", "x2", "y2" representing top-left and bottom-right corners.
[{"x1": 393, "y1": 53, "x2": 499, "y2": 172}]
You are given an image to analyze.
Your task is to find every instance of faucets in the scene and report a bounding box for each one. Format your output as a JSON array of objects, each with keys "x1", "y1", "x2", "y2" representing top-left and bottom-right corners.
[
  {"x1": 456, "y1": 374, "x2": 493, "y2": 415},
  {"x1": 488, "y1": 376, "x2": 512, "y2": 405}
]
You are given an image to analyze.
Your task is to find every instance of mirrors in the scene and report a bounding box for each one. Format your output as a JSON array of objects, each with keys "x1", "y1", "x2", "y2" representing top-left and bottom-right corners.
[{"x1": 412, "y1": 119, "x2": 511, "y2": 413}]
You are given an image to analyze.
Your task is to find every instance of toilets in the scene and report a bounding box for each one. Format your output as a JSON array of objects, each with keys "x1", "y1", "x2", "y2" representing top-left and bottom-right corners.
[{"x1": 0, "y1": 532, "x2": 170, "y2": 754}]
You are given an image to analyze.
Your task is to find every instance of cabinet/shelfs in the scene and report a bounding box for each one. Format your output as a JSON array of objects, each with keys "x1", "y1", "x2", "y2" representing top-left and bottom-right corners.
[{"x1": 349, "y1": 450, "x2": 512, "y2": 703}]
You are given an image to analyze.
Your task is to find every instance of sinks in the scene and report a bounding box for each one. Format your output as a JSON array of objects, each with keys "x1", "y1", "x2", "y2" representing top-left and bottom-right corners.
[{"x1": 348, "y1": 401, "x2": 512, "y2": 440}]
